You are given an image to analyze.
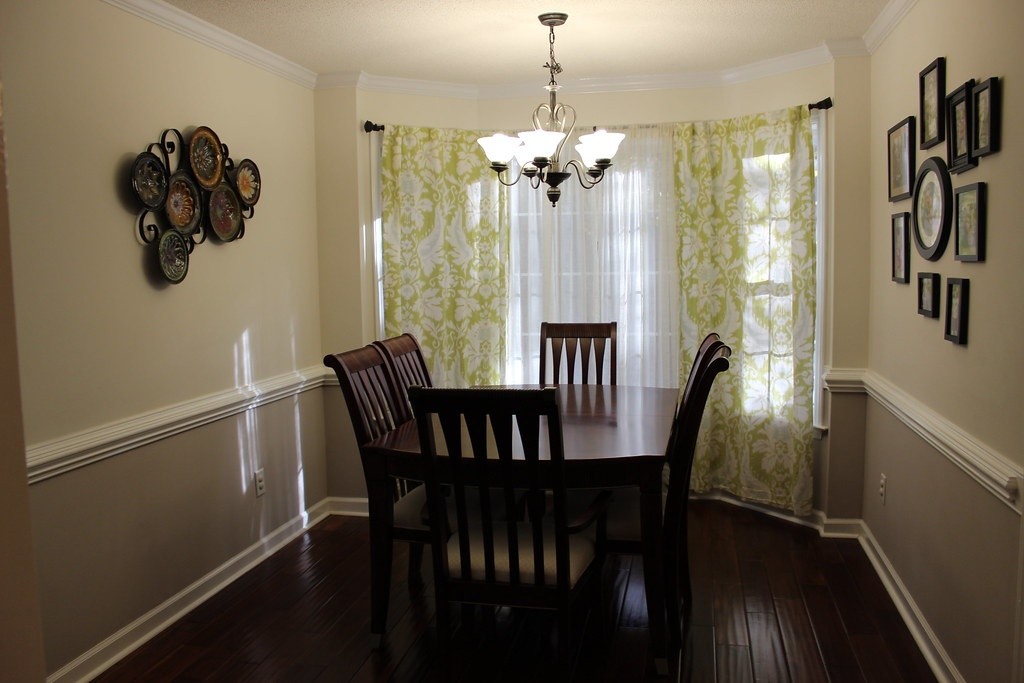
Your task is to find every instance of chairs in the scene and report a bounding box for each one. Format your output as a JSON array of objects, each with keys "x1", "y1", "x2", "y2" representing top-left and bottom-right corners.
[
  {"x1": 404, "y1": 382, "x2": 614, "y2": 682},
  {"x1": 521, "y1": 320, "x2": 617, "y2": 566},
  {"x1": 321, "y1": 342, "x2": 534, "y2": 662},
  {"x1": 673, "y1": 333, "x2": 725, "y2": 426},
  {"x1": 602, "y1": 343, "x2": 735, "y2": 653},
  {"x1": 374, "y1": 334, "x2": 534, "y2": 524}
]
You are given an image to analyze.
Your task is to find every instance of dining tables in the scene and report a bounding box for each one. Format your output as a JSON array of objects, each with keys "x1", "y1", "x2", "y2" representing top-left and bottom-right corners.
[{"x1": 360, "y1": 382, "x2": 681, "y2": 683}]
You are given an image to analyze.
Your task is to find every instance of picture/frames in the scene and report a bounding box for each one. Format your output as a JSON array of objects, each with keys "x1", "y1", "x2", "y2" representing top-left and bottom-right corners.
[{"x1": 887, "y1": 55, "x2": 1000, "y2": 345}]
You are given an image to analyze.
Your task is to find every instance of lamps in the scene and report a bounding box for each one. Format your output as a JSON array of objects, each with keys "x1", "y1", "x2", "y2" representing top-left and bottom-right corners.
[{"x1": 480, "y1": 12, "x2": 626, "y2": 208}]
